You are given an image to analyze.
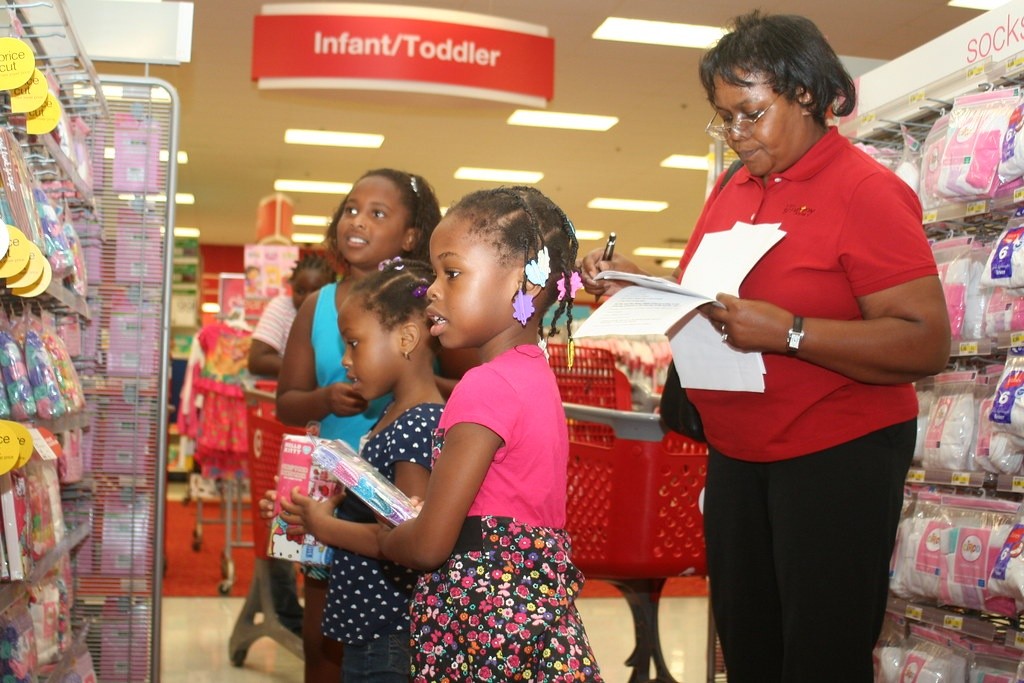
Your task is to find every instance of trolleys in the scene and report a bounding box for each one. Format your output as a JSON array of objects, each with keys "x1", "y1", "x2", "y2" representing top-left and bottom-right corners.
[
  {"x1": 545, "y1": 342, "x2": 714, "y2": 683},
  {"x1": 182, "y1": 325, "x2": 261, "y2": 550},
  {"x1": 229, "y1": 388, "x2": 324, "y2": 672}
]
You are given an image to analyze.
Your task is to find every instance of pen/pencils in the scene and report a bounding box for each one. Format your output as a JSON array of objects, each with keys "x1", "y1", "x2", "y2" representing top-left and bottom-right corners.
[{"x1": 594, "y1": 230, "x2": 618, "y2": 303}]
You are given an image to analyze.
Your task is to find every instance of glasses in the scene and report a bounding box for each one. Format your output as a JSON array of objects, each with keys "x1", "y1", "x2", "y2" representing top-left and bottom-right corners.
[{"x1": 704, "y1": 89, "x2": 790, "y2": 142}]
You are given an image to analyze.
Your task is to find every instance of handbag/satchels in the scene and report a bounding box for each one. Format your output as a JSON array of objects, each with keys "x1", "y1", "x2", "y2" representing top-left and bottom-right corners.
[{"x1": 659, "y1": 358, "x2": 706, "y2": 442}]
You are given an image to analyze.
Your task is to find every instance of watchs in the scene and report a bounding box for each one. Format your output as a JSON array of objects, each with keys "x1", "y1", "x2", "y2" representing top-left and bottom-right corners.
[{"x1": 784, "y1": 313, "x2": 805, "y2": 357}]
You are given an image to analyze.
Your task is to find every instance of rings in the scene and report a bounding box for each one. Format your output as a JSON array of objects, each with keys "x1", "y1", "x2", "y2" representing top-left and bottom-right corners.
[
  {"x1": 720, "y1": 323, "x2": 726, "y2": 332},
  {"x1": 721, "y1": 334, "x2": 728, "y2": 342}
]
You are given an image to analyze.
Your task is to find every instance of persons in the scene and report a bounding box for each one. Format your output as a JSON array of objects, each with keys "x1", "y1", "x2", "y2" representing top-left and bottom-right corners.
[
  {"x1": 274, "y1": 169, "x2": 486, "y2": 683},
  {"x1": 376, "y1": 185, "x2": 602, "y2": 683},
  {"x1": 258, "y1": 256, "x2": 446, "y2": 683},
  {"x1": 247, "y1": 253, "x2": 338, "y2": 377},
  {"x1": 577, "y1": 6, "x2": 952, "y2": 683}
]
